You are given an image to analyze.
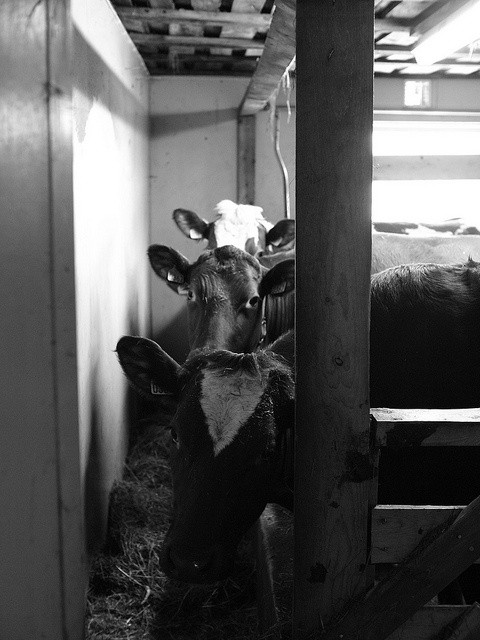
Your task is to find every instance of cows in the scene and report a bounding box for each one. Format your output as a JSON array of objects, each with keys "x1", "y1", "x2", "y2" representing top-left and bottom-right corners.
[
  {"x1": 173, "y1": 200, "x2": 480, "y2": 273},
  {"x1": 148, "y1": 245, "x2": 295, "y2": 352},
  {"x1": 115, "y1": 257, "x2": 480, "y2": 605}
]
